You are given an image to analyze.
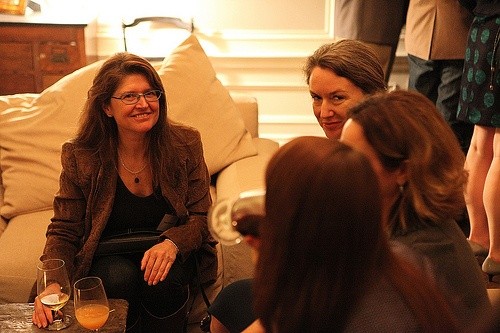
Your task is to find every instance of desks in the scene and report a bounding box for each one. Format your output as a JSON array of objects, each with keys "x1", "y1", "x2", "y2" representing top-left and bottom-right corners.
[{"x1": 0, "y1": 299, "x2": 128, "y2": 333}]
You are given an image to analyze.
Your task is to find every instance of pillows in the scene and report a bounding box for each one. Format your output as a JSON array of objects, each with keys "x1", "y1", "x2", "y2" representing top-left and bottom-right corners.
[{"x1": 0, "y1": 34, "x2": 258, "y2": 220}]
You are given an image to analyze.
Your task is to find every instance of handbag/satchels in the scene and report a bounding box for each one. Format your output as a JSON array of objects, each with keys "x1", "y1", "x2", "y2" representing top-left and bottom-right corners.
[{"x1": 89, "y1": 230, "x2": 189, "y2": 301}]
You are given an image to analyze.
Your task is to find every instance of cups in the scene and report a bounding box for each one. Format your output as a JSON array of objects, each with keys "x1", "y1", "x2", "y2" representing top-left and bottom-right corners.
[{"x1": 74, "y1": 277, "x2": 110, "y2": 333}]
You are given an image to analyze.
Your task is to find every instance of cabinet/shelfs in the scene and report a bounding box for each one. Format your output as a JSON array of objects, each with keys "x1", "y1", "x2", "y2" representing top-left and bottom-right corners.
[{"x1": 0, "y1": 22, "x2": 88, "y2": 94}]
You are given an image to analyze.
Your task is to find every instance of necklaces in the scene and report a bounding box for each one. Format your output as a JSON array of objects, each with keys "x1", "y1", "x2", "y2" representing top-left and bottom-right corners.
[{"x1": 116, "y1": 148, "x2": 150, "y2": 184}]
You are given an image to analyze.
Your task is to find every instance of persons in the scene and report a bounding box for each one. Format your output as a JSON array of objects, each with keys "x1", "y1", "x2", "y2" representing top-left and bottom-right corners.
[
  {"x1": 207, "y1": 40, "x2": 389, "y2": 333},
  {"x1": 339, "y1": 89, "x2": 493, "y2": 333},
  {"x1": 405, "y1": 0, "x2": 476, "y2": 156},
  {"x1": 238, "y1": 135, "x2": 464, "y2": 333},
  {"x1": 334, "y1": 0, "x2": 410, "y2": 83},
  {"x1": 26, "y1": 51, "x2": 220, "y2": 333},
  {"x1": 456, "y1": 0, "x2": 500, "y2": 275}
]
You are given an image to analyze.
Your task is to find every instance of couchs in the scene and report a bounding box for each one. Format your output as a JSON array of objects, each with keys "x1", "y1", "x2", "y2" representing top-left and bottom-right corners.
[{"x1": 1, "y1": 96, "x2": 280, "y2": 333}]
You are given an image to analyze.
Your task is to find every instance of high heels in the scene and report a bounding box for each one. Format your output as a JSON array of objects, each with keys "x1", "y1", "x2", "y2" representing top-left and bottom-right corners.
[
  {"x1": 481, "y1": 256, "x2": 500, "y2": 282},
  {"x1": 467, "y1": 239, "x2": 489, "y2": 258}
]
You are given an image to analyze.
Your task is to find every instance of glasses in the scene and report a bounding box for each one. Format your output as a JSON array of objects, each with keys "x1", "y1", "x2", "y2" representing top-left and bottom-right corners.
[{"x1": 110, "y1": 88, "x2": 163, "y2": 104}]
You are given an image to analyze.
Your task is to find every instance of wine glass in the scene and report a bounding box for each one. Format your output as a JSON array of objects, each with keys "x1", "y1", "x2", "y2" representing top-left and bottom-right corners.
[
  {"x1": 37, "y1": 259, "x2": 73, "y2": 331},
  {"x1": 207, "y1": 188, "x2": 267, "y2": 246}
]
